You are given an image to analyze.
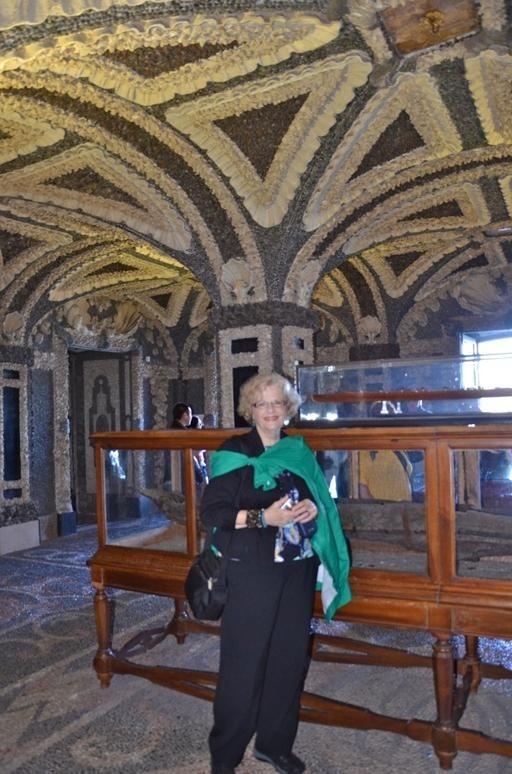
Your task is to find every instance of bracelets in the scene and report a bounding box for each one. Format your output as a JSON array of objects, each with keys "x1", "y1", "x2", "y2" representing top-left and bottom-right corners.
[{"x1": 246, "y1": 506, "x2": 267, "y2": 530}]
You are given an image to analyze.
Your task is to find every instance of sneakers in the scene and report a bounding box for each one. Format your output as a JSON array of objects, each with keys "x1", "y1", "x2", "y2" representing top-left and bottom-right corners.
[{"x1": 253, "y1": 746, "x2": 305, "y2": 773}]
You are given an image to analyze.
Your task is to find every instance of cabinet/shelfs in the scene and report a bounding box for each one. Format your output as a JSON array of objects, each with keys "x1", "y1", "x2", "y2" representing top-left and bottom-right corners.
[{"x1": 81, "y1": 420, "x2": 512, "y2": 774}]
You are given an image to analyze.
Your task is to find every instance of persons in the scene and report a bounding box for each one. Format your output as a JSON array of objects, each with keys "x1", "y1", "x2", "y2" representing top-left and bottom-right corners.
[
  {"x1": 167, "y1": 403, "x2": 218, "y2": 502},
  {"x1": 355, "y1": 382, "x2": 484, "y2": 416},
  {"x1": 199, "y1": 369, "x2": 327, "y2": 771}
]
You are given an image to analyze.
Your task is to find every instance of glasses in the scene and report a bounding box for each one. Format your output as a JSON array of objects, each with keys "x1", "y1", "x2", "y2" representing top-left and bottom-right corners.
[{"x1": 253, "y1": 399, "x2": 286, "y2": 408}]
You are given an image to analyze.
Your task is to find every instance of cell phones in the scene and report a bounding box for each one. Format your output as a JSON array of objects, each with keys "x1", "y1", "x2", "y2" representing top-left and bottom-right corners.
[{"x1": 296, "y1": 519, "x2": 317, "y2": 539}]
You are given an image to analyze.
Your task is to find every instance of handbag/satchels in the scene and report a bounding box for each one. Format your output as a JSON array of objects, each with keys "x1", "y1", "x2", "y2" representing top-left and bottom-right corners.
[{"x1": 183, "y1": 434, "x2": 249, "y2": 620}]
